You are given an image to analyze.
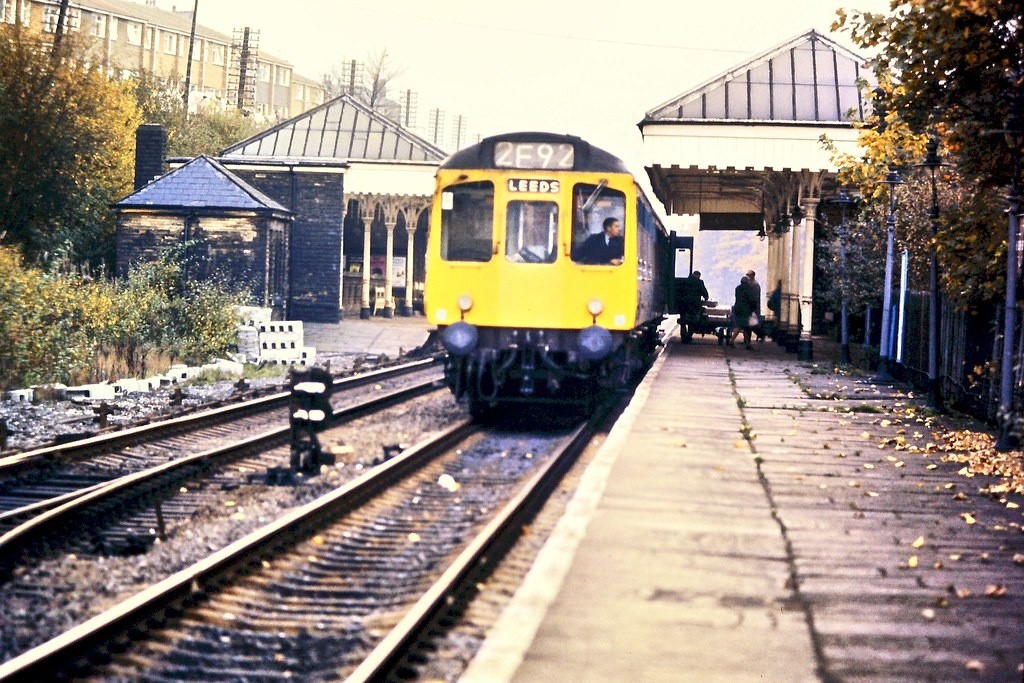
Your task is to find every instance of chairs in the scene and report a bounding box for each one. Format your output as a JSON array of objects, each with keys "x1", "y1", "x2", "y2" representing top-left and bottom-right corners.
[{"x1": 372, "y1": 285, "x2": 396, "y2": 316}]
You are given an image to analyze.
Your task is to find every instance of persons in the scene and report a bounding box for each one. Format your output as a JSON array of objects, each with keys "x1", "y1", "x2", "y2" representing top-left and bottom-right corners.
[
  {"x1": 768, "y1": 280, "x2": 782, "y2": 328},
  {"x1": 680, "y1": 271, "x2": 709, "y2": 344},
  {"x1": 728, "y1": 270, "x2": 761, "y2": 352},
  {"x1": 573, "y1": 217, "x2": 625, "y2": 266}
]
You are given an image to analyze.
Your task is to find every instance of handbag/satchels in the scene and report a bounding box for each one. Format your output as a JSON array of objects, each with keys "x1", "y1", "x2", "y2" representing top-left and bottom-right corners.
[{"x1": 767, "y1": 299, "x2": 777, "y2": 311}]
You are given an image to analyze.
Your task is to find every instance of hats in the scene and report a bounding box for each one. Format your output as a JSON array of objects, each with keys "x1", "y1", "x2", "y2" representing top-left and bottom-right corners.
[{"x1": 746, "y1": 269, "x2": 755, "y2": 276}]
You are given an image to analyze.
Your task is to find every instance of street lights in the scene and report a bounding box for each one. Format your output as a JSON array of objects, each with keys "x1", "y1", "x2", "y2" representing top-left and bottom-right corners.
[
  {"x1": 875, "y1": 162, "x2": 907, "y2": 370},
  {"x1": 829, "y1": 187, "x2": 857, "y2": 364},
  {"x1": 912, "y1": 138, "x2": 952, "y2": 409},
  {"x1": 985, "y1": 114, "x2": 1023, "y2": 413}
]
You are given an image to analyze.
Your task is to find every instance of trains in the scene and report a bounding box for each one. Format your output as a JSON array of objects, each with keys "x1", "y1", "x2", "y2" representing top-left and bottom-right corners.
[{"x1": 423, "y1": 131, "x2": 669, "y2": 424}]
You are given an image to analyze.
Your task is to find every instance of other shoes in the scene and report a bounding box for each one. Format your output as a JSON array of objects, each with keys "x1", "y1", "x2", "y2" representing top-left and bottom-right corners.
[
  {"x1": 680, "y1": 334, "x2": 695, "y2": 344},
  {"x1": 729, "y1": 339, "x2": 735, "y2": 347},
  {"x1": 745, "y1": 344, "x2": 754, "y2": 351}
]
